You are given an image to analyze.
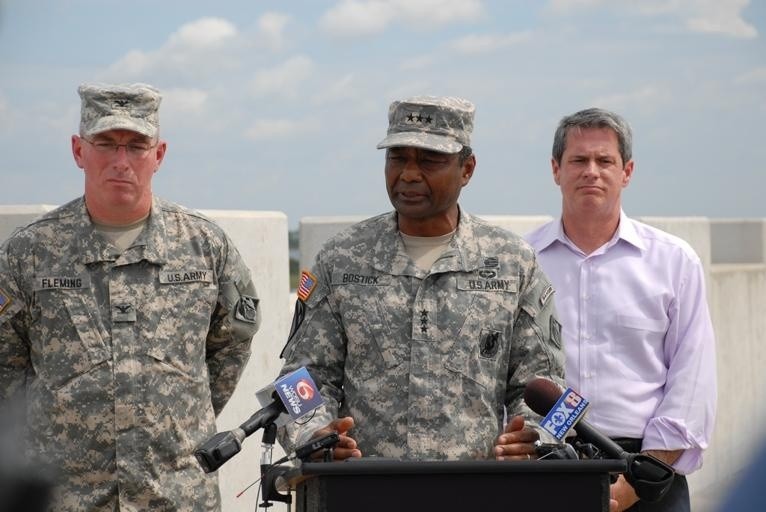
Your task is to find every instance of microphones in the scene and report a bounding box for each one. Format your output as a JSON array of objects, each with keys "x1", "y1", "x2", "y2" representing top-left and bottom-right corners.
[
  {"x1": 523, "y1": 378, "x2": 675, "y2": 503},
  {"x1": 194, "y1": 366, "x2": 326, "y2": 473}
]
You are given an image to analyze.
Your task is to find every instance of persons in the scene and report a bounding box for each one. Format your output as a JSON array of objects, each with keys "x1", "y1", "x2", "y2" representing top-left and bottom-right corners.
[
  {"x1": 276, "y1": 94, "x2": 566, "y2": 463},
  {"x1": 0, "y1": 84, "x2": 262, "y2": 512},
  {"x1": 524, "y1": 107, "x2": 716, "y2": 512}
]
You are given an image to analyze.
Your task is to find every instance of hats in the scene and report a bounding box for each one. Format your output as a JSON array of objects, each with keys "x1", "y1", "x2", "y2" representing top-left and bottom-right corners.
[
  {"x1": 78, "y1": 83, "x2": 162, "y2": 138},
  {"x1": 376, "y1": 96, "x2": 474, "y2": 154}
]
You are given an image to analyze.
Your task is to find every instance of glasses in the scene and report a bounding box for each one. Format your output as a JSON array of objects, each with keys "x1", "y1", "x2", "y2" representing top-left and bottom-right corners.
[{"x1": 81, "y1": 137, "x2": 158, "y2": 159}]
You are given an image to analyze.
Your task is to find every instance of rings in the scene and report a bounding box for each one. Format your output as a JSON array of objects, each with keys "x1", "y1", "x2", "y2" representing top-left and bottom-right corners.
[{"x1": 527, "y1": 453, "x2": 531, "y2": 461}]
